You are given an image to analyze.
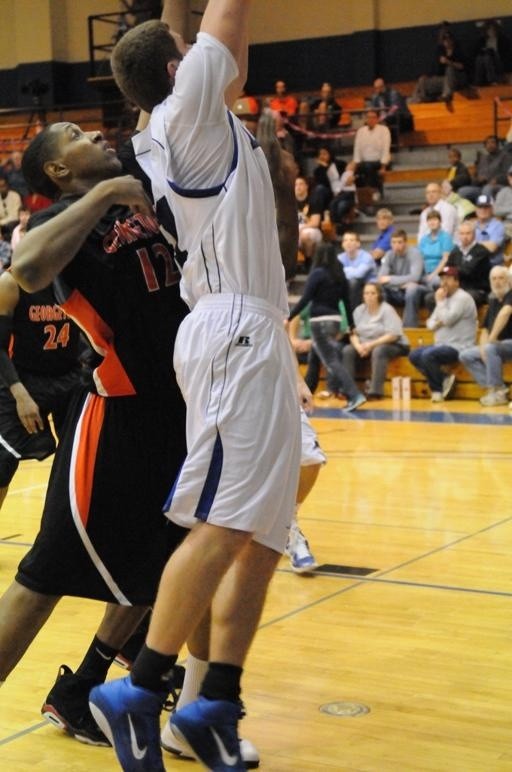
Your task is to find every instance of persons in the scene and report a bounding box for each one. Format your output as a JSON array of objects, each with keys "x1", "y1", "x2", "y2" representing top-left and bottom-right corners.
[
  {"x1": 2, "y1": 152, "x2": 53, "y2": 252},
  {"x1": 438, "y1": 21, "x2": 506, "y2": 101},
  {"x1": 283, "y1": 321, "x2": 324, "y2": 578},
  {"x1": 270, "y1": 76, "x2": 413, "y2": 271},
  {"x1": 0, "y1": 0, "x2": 203, "y2": 748},
  {"x1": 111, "y1": 609, "x2": 187, "y2": 690},
  {"x1": 290, "y1": 208, "x2": 433, "y2": 409},
  {"x1": 1, "y1": 217, "x2": 90, "y2": 511},
  {"x1": 158, "y1": 604, "x2": 261, "y2": 770},
  {"x1": 409, "y1": 136, "x2": 510, "y2": 409},
  {"x1": 91, "y1": 0, "x2": 326, "y2": 772}
]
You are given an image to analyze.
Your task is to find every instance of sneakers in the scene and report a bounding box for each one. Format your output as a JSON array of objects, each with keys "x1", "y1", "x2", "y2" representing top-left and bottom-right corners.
[
  {"x1": 285, "y1": 524, "x2": 317, "y2": 573},
  {"x1": 315, "y1": 376, "x2": 512, "y2": 413},
  {"x1": 42, "y1": 635, "x2": 259, "y2": 772}
]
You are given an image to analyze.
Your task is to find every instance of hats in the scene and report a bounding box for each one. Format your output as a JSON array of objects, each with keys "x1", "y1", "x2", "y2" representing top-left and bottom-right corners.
[
  {"x1": 439, "y1": 267, "x2": 458, "y2": 276},
  {"x1": 476, "y1": 195, "x2": 493, "y2": 207}
]
